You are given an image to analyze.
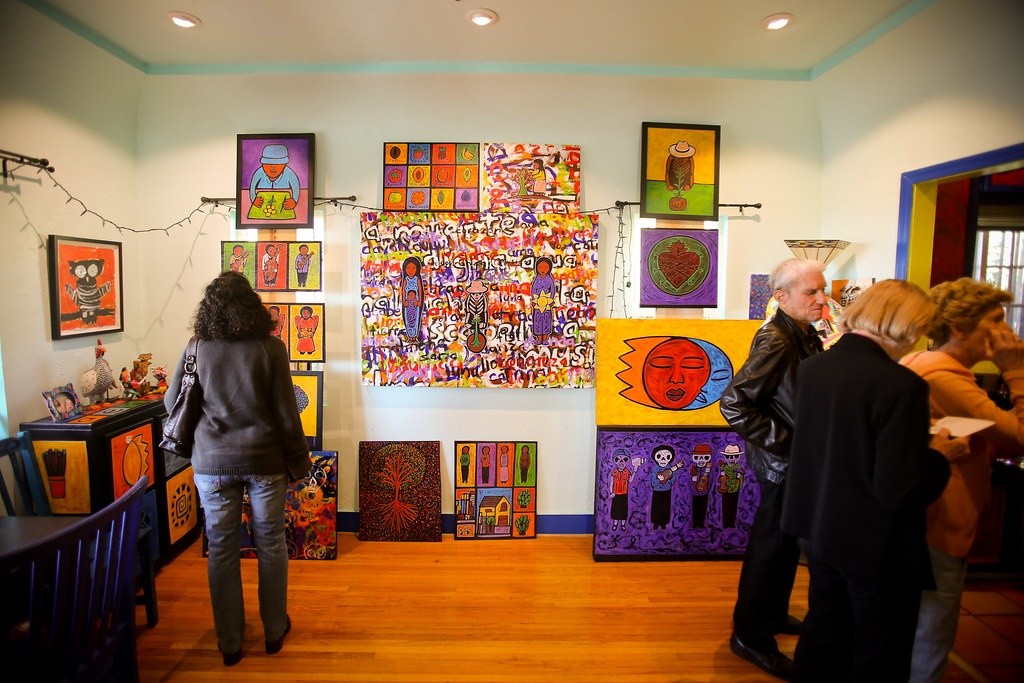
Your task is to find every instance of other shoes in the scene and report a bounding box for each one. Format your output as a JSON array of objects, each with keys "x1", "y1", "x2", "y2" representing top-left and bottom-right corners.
[
  {"x1": 265, "y1": 613, "x2": 291, "y2": 653},
  {"x1": 218, "y1": 641, "x2": 243, "y2": 666}
]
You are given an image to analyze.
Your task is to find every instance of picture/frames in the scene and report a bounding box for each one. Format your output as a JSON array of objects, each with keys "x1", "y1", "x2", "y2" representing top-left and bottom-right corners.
[
  {"x1": 47, "y1": 232, "x2": 124, "y2": 340},
  {"x1": 383, "y1": 142, "x2": 480, "y2": 212},
  {"x1": 261, "y1": 302, "x2": 326, "y2": 363},
  {"x1": 41, "y1": 382, "x2": 86, "y2": 423},
  {"x1": 451, "y1": 438, "x2": 539, "y2": 541},
  {"x1": 233, "y1": 132, "x2": 316, "y2": 230},
  {"x1": 592, "y1": 428, "x2": 762, "y2": 563},
  {"x1": 638, "y1": 120, "x2": 721, "y2": 222},
  {"x1": 289, "y1": 367, "x2": 325, "y2": 451},
  {"x1": 220, "y1": 239, "x2": 324, "y2": 296}
]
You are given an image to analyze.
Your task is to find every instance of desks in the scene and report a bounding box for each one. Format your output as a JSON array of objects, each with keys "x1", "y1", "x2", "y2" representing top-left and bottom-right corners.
[{"x1": 0, "y1": 514, "x2": 90, "y2": 567}]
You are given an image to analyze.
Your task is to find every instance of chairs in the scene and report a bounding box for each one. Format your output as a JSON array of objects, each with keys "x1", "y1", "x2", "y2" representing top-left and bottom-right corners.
[
  {"x1": 0, "y1": 474, "x2": 151, "y2": 683},
  {"x1": 0, "y1": 430, "x2": 159, "y2": 629}
]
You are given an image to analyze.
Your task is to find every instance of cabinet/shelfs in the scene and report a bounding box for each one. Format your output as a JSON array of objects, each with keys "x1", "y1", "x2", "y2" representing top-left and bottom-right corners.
[{"x1": 19, "y1": 385, "x2": 205, "y2": 594}]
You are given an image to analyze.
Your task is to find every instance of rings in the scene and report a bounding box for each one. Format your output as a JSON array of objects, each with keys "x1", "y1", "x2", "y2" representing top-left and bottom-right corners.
[{"x1": 1015, "y1": 338, "x2": 1021, "y2": 341}]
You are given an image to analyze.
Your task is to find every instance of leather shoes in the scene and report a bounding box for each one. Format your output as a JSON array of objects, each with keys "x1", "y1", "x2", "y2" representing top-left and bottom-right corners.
[
  {"x1": 733, "y1": 610, "x2": 805, "y2": 635},
  {"x1": 729, "y1": 629, "x2": 794, "y2": 680}
]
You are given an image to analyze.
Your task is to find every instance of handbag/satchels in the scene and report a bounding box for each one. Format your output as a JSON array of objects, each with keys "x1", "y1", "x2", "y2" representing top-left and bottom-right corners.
[{"x1": 160, "y1": 335, "x2": 204, "y2": 458}]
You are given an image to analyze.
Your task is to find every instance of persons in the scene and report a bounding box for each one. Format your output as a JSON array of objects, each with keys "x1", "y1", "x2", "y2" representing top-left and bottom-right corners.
[
  {"x1": 164, "y1": 272, "x2": 312, "y2": 665},
  {"x1": 718, "y1": 258, "x2": 827, "y2": 680},
  {"x1": 897, "y1": 280, "x2": 1024, "y2": 683},
  {"x1": 64, "y1": 398, "x2": 75, "y2": 412},
  {"x1": 781, "y1": 278, "x2": 971, "y2": 683}
]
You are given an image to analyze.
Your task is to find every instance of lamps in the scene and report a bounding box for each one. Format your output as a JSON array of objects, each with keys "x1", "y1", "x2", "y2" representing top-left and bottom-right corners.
[{"x1": 782, "y1": 237, "x2": 854, "y2": 272}]
